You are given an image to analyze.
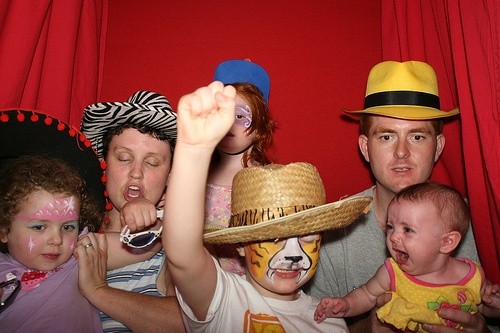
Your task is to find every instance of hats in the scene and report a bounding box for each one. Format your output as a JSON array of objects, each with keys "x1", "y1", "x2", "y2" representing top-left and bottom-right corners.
[
  {"x1": 0, "y1": 108, "x2": 113, "y2": 234},
  {"x1": 203, "y1": 161, "x2": 373, "y2": 246},
  {"x1": 214, "y1": 58, "x2": 270, "y2": 101},
  {"x1": 80, "y1": 90, "x2": 177, "y2": 156},
  {"x1": 344, "y1": 61, "x2": 459, "y2": 123}
]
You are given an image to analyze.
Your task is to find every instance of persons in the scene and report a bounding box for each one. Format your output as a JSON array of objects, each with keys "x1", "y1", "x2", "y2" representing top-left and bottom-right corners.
[{"x1": 0, "y1": 60, "x2": 500, "y2": 333}]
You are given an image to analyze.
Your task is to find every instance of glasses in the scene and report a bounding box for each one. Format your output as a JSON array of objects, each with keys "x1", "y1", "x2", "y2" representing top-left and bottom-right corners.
[
  {"x1": 120, "y1": 217, "x2": 163, "y2": 249},
  {"x1": 0, "y1": 278, "x2": 22, "y2": 314}
]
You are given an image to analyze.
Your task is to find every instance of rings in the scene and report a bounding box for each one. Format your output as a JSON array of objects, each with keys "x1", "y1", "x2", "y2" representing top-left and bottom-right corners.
[{"x1": 85, "y1": 243, "x2": 92, "y2": 249}]
